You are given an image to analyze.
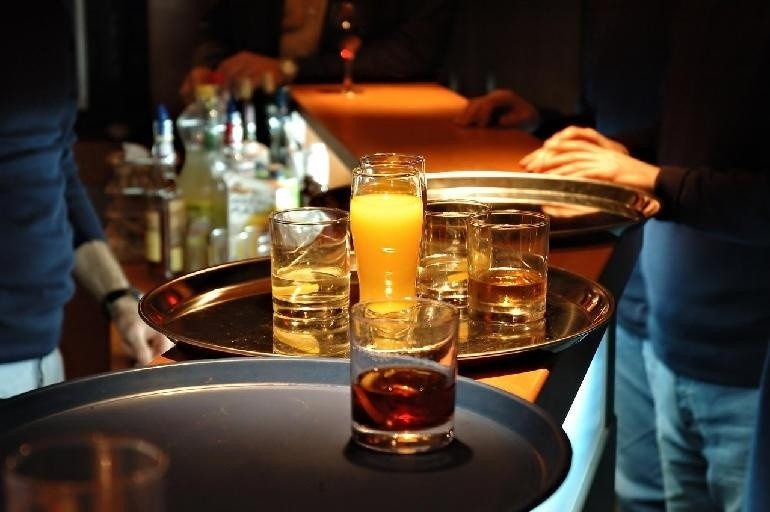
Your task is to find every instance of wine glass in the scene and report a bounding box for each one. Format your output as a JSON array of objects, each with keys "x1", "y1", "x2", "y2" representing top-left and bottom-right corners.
[{"x1": 335, "y1": 21, "x2": 363, "y2": 94}]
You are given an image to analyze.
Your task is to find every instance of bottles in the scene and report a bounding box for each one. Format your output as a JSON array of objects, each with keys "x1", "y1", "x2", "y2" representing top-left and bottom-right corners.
[{"x1": 144, "y1": 86, "x2": 320, "y2": 280}]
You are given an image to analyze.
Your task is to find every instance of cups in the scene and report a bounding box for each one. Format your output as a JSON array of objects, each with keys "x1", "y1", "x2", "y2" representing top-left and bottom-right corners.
[
  {"x1": 350, "y1": 165, "x2": 423, "y2": 323},
  {"x1": 465, "y1": 210, "x2": 549, "y2": 324},
  {"x1": 270, "y1": 206, "x2": 351, "y2": 324},
  {"x1": 349, "y1": 297, "x2": 460, "y2": 455},
  {"x1": 359, "y1": 155, "x2": 427, "y2": 210},
  {"x1": 418, "y1": 200, "x2": 490, "y2": 309}
]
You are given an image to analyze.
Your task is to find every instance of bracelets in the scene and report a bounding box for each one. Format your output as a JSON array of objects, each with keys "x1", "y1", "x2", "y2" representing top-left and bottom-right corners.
[{"x1": 98, "y1": 287, "x2": 145, "y2": 312}]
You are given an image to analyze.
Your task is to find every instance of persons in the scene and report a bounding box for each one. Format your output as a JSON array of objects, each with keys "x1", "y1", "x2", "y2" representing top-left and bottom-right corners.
[
  {"x1": 173, "y1": 2, "x2": 453, "y2": 121},
  {"x1": 510, "y1": 0, "x2": 768, "y2": 510},
  {"x1": 0, "y1": 1, "x2": 181, "y2": 406},
  {"x1": 452, "y1": 4, "x2": 675, "y2": 165}
]
